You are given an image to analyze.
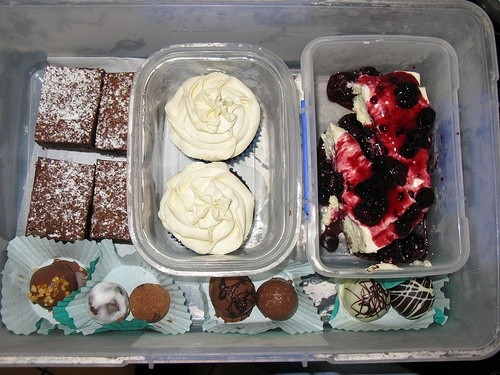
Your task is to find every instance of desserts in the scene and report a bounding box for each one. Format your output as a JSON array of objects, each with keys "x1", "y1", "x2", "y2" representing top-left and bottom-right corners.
[
  {"x1": 386, "y1": 277, "x2": 435, "y2": 321},
  {"x1": 163, "y1": 71, "x2": 265, "y2": 162},
  {"x1": 26, "y1": 259, "x2": 171, "y2": 325},
  {"x1": 209, "y1": 276, "x2": 256, "y2": 323},
  {"x1": 157, "y1": 161, "x2": 255, "y2": 258},
  {"x1": 338, "y1": 278, "x2": 390, "y2": 323},
  {"x1": 25, "y1": 157, "x2": 135, "y2": 244},
  {"x1": 32, "y1": 65, "x2": 135, "y2": 159},
  {"x1": 316, "y1": 66, "x2": 439, "y2": 271},
  {"x1": 255, "y1": 276, "x2": 300, "y2": 323}
]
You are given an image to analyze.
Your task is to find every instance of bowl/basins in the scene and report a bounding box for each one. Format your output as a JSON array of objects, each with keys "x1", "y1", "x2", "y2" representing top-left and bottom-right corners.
[
  {"x1": 299, "y1": 34, "x2": 471, "y2": 281},
  {"x1": 125, "y1": 38, "x2": 300, "y2": 278}
]
